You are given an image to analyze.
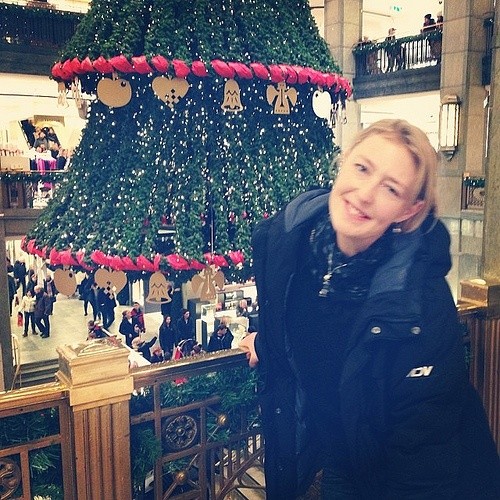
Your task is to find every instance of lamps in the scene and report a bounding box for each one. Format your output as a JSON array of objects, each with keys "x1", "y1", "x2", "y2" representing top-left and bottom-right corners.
[{"x1": 440, "y1": 94, "x2": 460, "y2": 152}]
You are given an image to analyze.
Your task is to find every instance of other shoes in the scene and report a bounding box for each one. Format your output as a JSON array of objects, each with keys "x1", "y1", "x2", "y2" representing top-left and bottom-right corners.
[
  {"x1": 22, "y1": 333, "x2": 29, "y2": 337},
  {"x1": 41, "y1": 334, "x2": 50, "y2": 338},
  {"x1": 40, "y1": 330, "x2": 47, "y2": 336},
  {"x1": 32, "y1": 330, "x2": 38, "y2": 335}
]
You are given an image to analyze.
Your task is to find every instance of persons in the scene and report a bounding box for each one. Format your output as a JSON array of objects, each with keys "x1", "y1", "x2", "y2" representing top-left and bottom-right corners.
[
  {"x1": 238, "y1": 118, "x2": 499, "y2": 500},
  {"x1": 23, "y1": 119, "x2": 76, "y2": 170},
  {"x1": 7, "y1": 245, "x2": 261, "y2": 368},
  {"x1": 356, "y1": 12, "x2": 444, "y2": 75}
]
